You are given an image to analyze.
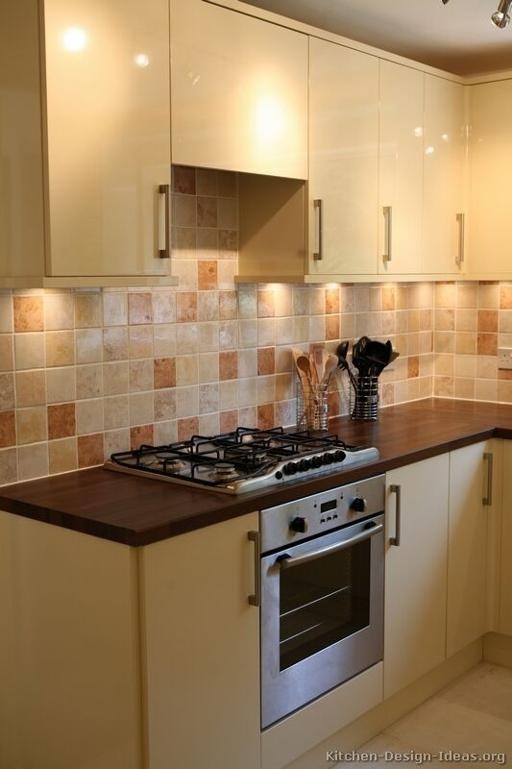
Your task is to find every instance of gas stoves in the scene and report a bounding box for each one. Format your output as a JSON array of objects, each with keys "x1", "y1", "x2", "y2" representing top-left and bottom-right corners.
[{"x1": 102, "y1": 425, "x2": 382, "y2": 496}]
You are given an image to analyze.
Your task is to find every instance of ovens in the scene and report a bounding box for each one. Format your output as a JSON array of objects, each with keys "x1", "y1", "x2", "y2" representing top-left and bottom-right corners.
[{"x1": 258, "y1": 473, "x2": 388, "y2": 731}]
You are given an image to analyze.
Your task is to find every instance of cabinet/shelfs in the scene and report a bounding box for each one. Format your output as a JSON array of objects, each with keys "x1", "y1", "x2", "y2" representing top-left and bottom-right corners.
[
  {"x1": 0, "y1": 0, "x2": 170, "y2": 287},
  {"x1": 470, "y1": 75, "x2": 512, "y2": 282},
  {"x1": 0, "y1": 512, "x2": 261, "y2": 769},
  {"x1": 384, "y1": 437, "x2": 512, "y2": 730},
  {"x1": 235, "y1": 36, "x2": 469, "y2": 283},
  {"x1": 170, "y1": 1, "x2": 308, "y2": 182}
]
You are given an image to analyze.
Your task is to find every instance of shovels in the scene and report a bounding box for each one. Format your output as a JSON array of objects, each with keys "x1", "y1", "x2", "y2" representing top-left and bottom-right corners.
[{"x1": 337, "y1": 341, "x2": 357, "y2": 391}]
